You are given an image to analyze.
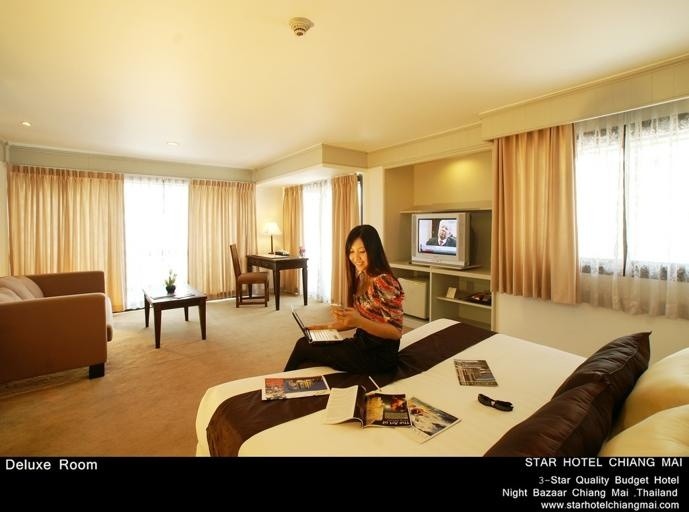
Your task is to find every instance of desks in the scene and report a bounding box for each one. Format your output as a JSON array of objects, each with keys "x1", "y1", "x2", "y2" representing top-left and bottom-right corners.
[{"x1": 246, "y1": 253, "x2": 310, "y2": 311}]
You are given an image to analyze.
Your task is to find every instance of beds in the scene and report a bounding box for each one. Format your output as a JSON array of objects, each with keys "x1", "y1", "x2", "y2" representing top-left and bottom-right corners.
[{"x1": 195, "y1": 318, "x2": 689, "y2": 457}]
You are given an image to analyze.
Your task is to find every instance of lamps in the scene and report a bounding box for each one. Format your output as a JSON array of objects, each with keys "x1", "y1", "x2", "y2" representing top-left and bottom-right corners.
[{"x1": 261, "y1": 222, "x2": 281, "y2": 254}]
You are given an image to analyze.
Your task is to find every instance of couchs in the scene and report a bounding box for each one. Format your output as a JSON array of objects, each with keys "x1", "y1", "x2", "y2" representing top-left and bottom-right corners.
[{"x1": 0, "y1": 270, "x2": 113, "y2": 385}]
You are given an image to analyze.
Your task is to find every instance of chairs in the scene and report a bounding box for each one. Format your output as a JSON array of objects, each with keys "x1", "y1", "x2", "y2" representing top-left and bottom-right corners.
[{"x1": 230, "y1": 244, "x2": 270, "y2": 308}]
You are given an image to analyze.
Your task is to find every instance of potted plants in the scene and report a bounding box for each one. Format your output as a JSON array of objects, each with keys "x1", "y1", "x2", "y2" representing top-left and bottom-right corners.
[{"x1": 164, "y1": 269, "x2": 178, "y2": 295}]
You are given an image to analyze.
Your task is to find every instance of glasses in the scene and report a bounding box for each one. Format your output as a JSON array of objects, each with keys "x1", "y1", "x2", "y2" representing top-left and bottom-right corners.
[{"x1": 478, "y1": 394, "x2": 513, "y2": 412}]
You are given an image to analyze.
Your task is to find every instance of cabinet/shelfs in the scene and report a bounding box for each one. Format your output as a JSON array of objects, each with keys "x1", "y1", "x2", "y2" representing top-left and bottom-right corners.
[{"x1": 389, "y1": 207, "x2": 496, "y2": 331}]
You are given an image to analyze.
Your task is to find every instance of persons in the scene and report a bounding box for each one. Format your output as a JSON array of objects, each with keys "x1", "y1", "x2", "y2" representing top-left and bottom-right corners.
[
  {"x1": 425, "y1": 222, "x2": 456, "y2": 247},
  {"x1": 282, "y1": 224, "x2": 405, "y2": 378}
]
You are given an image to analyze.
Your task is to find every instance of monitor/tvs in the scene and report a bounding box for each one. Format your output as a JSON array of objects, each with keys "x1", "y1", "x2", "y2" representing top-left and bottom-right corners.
[{"x1": 411, "y1": 213, "x2": 470, "y2": 267}]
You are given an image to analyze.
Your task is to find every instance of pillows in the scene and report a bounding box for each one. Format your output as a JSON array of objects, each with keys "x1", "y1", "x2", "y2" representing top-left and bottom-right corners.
[
  {"x1": 483, "y1": 383, "x2": 614, "y2": 457},
  {"x1": 620, "y1": 347, "x2": 689, "y2": 429},
  {"x1": 552, "y1": 330, "x2": 651, "y2": 423},
  {"x1": 597, "y1": 402, "x2": 689, "y2": 457}
]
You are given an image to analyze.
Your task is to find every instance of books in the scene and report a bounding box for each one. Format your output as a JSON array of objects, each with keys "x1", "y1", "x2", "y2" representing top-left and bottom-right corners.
[
  {"x1": 391, "y1": 395, "x2": 462, "y2": 445},
  {"x1": 260, "y1": 374, "x2": 330, "y2": 400},
  {"x1": 453, "y1": 358, "x2": 498, "y2": 387},
  {"x1": 322, "y1": 384, "x2": 413, "y2": 429}
]
